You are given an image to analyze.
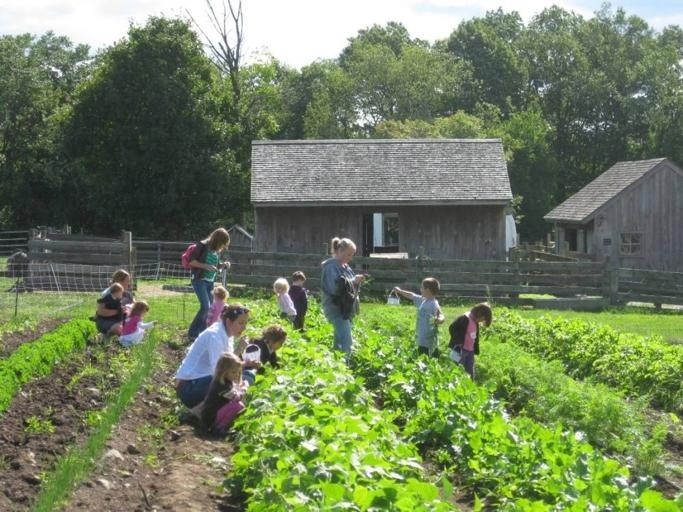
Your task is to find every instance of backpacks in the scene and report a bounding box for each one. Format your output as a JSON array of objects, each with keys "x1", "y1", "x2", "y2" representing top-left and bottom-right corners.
[{"x1": 181, "y1": 240, "x2": 206, "y2": 268}]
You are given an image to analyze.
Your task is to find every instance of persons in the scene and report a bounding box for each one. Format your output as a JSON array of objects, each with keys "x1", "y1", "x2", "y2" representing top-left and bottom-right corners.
[
  {"x1": 188, "y1": 228, "x2": 232, "y2": 343},
  {"x1": 205, "y1": 286, "x2": 230, "y2": 327},
  {"x1": 393, "y1": 277, "x2": 446, "y2": 359},
  {"x1": 319, "y1": 237, "x2": 366, "y2": 363},
  {"x1": 240, "y1": 325, "x2": 288, "y2": 384},
  {"x1": 118, "y1": 299, "x2": 158, "y2": 347},
  {"x1": 201, "y1": 351, "x2": 249, "y2": 435},
  {"x1": 95, "y1": 269, "x2": 131, "y2": 336},
  {"x1": 449, "y1": 302, "x2": 493, "y2": 383},
  {"x1": 273, "y1": 278, "x2": 298, "y2": 323},
  {"x1": 288, "y1": 270, "x2": 308, "y2": 330},
  {"x1": 96, "y1": 282, "x2": 125, "y2": 322},
  {"x1": 174, "y1": 302, "x2": 261, "y2": 422}
]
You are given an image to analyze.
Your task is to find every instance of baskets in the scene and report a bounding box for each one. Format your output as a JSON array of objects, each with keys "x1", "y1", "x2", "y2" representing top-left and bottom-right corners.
[
  {"x1": 242, "y1": 344, "x2": 261, "y2": 363},
  {"x1": 388, "y1": 289, "x2": 399, "y2": 305},
  {"x1": 450, "y1": 345, "x2": 462, "y2": 363}
]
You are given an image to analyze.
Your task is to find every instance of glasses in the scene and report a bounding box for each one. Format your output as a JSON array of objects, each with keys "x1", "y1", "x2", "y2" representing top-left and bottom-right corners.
[{"x1": 233, "y1": 308, "x2": 250, "y2": 315}]
